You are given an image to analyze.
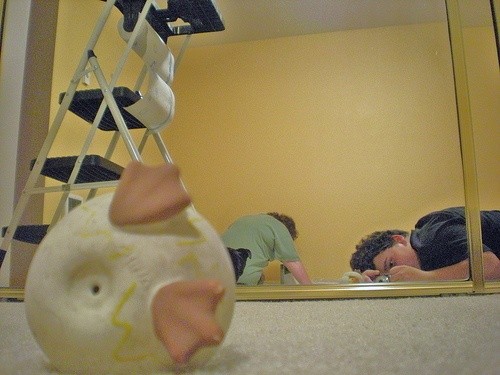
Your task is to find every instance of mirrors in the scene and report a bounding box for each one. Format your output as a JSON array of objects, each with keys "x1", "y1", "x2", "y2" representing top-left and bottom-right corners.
[{"x1": 0, "y1": 0, "x2": 500, "y2": 298}]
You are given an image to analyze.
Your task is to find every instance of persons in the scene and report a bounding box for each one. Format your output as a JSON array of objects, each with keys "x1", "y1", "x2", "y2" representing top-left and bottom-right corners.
[
  {"x1": 219, "y1": 212, "x2": 312, "y2": 286},
  {"x1": 349, "y1": 206, "x2": 500, "y2": 280}
]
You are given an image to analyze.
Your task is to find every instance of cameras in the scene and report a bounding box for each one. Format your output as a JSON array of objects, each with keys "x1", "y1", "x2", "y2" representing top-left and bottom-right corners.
[{"x1": 374, "y1": 273, "x2": 391, "y2": 283}]
